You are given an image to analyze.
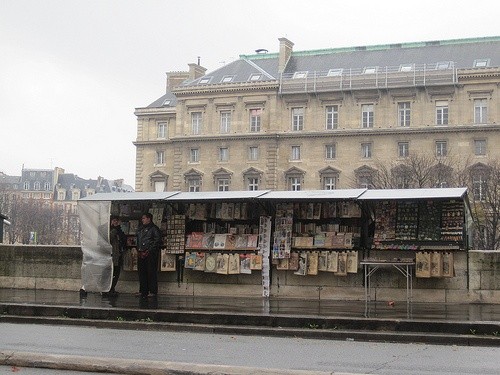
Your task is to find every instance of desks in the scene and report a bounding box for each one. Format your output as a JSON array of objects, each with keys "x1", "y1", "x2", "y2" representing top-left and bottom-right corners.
[{"x1": 360, "y1": 261, "x2": 415, "y2": 306}]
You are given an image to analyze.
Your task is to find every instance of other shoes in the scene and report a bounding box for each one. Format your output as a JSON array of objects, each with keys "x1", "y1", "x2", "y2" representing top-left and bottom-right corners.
[
  {"x1": 142, "y1": 293, "x2": 148, "y2": 299},
  {"x1": 103, "y1": 291, "x2": 117, "y2": 297},
  {"x1": 134, "y1": 291, "x2": 143, "y2": 296},
  {"x1": 147, "y1": 292, "x2": 156, "y2": 297}
]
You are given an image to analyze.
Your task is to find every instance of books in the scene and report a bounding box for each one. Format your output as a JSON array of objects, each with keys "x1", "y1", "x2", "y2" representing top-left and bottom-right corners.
[{"x1": 110, "y1": 201, "x2": 362, "y2": 276}]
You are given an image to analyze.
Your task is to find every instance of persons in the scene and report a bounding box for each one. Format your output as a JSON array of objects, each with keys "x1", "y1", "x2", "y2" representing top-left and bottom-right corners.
[
  {"x1": 134, "y1": 212, "x2": 162, "y2": 297},
  {"x1": 98, "y1": 215, "x2": 127, "y2": 297}
]
